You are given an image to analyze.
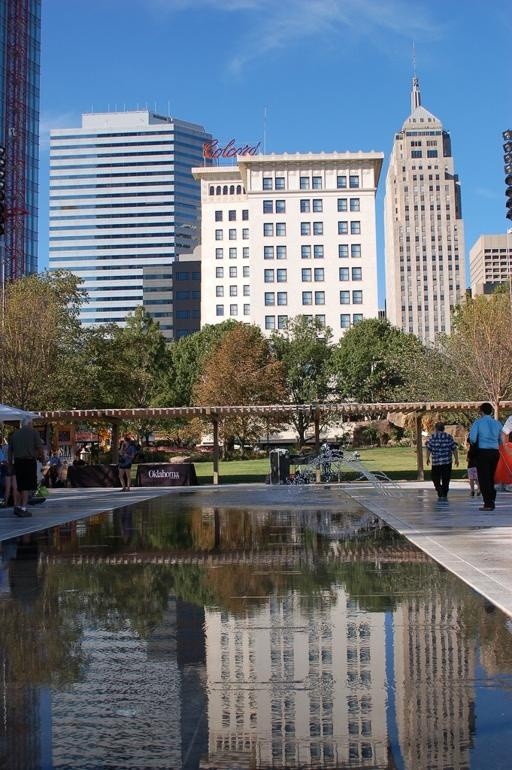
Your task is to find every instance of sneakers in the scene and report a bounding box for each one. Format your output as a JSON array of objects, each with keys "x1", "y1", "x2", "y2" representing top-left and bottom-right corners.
[
  {"x1": 470, "y1": 491, "x2": 481, "y2": 496},
  {"x1": 14, "y1": 507, "x2": 32, "y2": 517},
  {"x1": 438, "y1": 488, "x2": 447, "y2": 497}
]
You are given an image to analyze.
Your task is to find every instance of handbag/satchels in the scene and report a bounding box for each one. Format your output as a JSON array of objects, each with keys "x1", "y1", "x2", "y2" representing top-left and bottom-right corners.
[{"x1": 494, "y1": 442, "x2": 512, "y2": 484}]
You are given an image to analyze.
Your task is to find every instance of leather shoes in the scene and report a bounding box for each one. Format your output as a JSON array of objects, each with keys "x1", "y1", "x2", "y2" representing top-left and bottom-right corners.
[{"x1": 479, "y1": 507, "x2": 493, "y2": 511}]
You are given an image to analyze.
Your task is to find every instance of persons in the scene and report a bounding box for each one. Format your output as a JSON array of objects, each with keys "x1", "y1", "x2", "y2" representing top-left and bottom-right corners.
[
  {"x1": 117, "y1": 431, "x2": 141, "y2": 492},
  {"x1": 469, "y1": 402, "x2": 503, "y2": 511},
  {"x1": 8, "y1": 534, "x2": 49, "y2": 615},
  {"x1": 119, "y1": 506, "x2": 133, "y2": 546},
  {"x1": 500, "y1": 414, "x2": 512, "y2": 443},
  {"x1": 41, "y1": 442, "x2": 96, "y2": 488},
  {"x1": 465, "y1": 437, "x2": 482, "y2": 497},
  {"x1": 0, "y1": 538, "x2": 20, "y2": 601},
  {"x1": 320, "y1": 439, "x2": 331, "y2": 482},
  {"x1": 425, "y1": 421, "x2": 460, "y2": 497},
  {"x1": 7, "y1": 415, "x2": 44, "y2": 516},
  {"x1": 0, "y1": 431, "x2": 17, "y2": 507}
]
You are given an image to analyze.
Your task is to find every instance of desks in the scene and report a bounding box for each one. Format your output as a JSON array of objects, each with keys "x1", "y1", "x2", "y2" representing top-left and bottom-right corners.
[
  {"x1": 68, "y1": 464, "x2": 119, "y2": 487},
  {"x1": 137, "y1": 464, "x2": 196, "y2": 486}
]
